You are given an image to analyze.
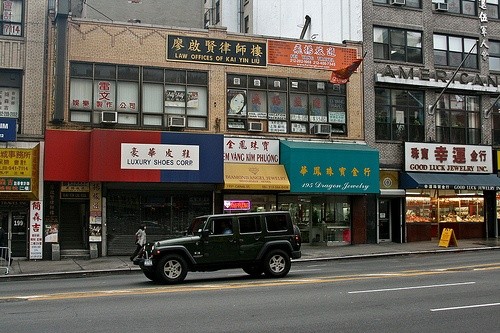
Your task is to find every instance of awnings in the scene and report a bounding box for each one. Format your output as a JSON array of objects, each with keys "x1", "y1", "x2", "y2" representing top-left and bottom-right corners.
[
  {"x1": 405, "y1": 173, "x2": 500, "y2": 190},
  {"x1": 224, "y1": 163, "x2": 290, "y2": 190}
]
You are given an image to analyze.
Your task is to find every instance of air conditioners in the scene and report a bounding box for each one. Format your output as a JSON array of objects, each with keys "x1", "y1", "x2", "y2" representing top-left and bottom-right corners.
[
  {"x1": 248, "y1": 122, "x2": 263, "y2": 132},
  {"x1": 168, "y1": 117, "x2": 186, "y2": 129},
  {"x1": 435, "y1": 2, "x2": 448, "y2": 11},
  {"x1": 314, "y1": 124, "x2": 332, "y2": 135},
  {"x1": 100, "y1": 111, "x2": 118, "y2": 124},
  {"x1": 390, "y1": 0, "x2": 406, "y2": 5}
]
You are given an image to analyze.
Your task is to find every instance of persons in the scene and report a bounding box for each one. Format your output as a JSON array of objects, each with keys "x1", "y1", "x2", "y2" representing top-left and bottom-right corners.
[
  {"x1": 129, "y1": 224, "x2": 147, "y2": 262},
  {"x1": 227, "y1": 90, "x2": 247, "y2": 115}
]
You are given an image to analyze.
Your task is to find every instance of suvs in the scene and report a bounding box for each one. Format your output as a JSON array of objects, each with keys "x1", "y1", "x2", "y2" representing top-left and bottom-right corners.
[{"x1": 131, "y1": 210, "x2": 302, "y2": 284}]
